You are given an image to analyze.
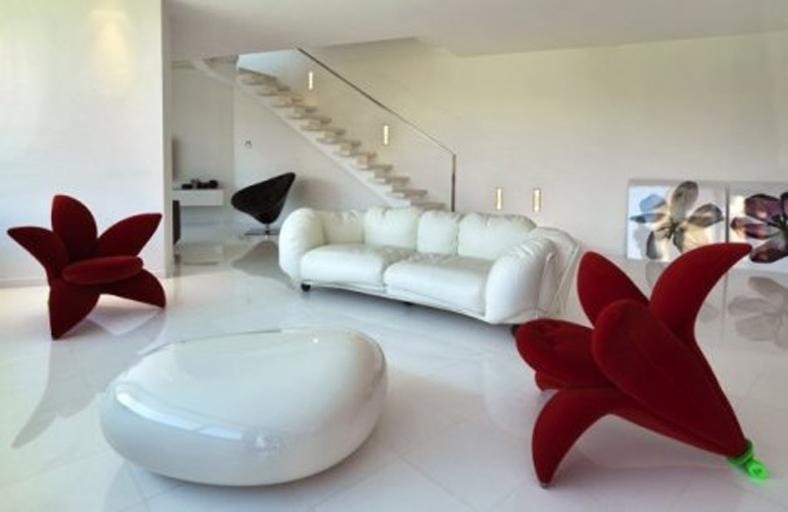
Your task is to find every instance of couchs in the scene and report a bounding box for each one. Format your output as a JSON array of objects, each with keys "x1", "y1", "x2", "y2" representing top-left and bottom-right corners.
[
  {"x1": 278, "y1": 206, "x2": 579, "y2": 325},
  {"x1": 7, "y1": 193, "x2": 167, "y2": 342},
  {"x1": 513, "y1": 242, "x2": 769, "y2": 489}
]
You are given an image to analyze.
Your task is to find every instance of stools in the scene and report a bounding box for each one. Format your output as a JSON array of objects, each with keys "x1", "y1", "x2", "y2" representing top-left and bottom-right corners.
[{"x1": 231, "y1": 172, "x2": 296, "y2": 237}]
